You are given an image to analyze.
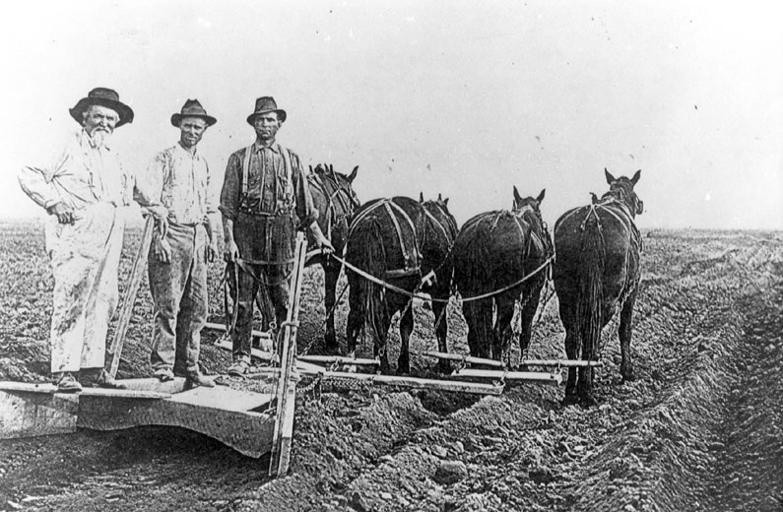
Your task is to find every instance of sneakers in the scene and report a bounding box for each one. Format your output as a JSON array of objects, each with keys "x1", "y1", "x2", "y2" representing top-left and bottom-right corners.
[{"x1": 227, "y1": 361, "x2": 250, "y2": 376}]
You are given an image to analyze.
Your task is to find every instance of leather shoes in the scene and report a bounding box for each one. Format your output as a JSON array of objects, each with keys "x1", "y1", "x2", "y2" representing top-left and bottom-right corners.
[
  {"x1": 51, "y1": 372, "x2": 82, "y2": 391},
  {"x1": 80, "y1": 370, "x2": 126, "y2": 390},
  {"x1": 154, "y1": 368, "x2": 173, "y2": 380},
  {"x1": 175, "y1": 368, "x2": 216, "y2": 389}
]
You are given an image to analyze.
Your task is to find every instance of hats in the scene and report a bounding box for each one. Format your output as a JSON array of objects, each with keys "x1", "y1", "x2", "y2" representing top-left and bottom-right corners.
[
  {"x1": 68, "y1": 86, "x2": 134, "y2": 128},
  {"x1": 247, "y1": 96, "x2": 286, "y2": 125},
  {"x1": 170, "y1": 99, "x2": 216, "y2": 128}
]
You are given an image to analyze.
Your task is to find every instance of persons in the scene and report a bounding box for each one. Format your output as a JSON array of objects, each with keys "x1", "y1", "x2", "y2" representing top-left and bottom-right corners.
[
  {"x1": 132, "y1": 96, "x2": 221, "y2": 387},
  {"x1": 212, "y1": 96, "x2": 335, "y2": 379},
  {"x1": 14, "y1": 86, "x2": 167, "y2": 396}
]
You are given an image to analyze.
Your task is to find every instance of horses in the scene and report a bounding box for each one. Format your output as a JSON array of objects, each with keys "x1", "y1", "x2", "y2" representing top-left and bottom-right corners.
[
  {"x1": 348, "y1": 191, "x2": 455, "y2": 385},
  {"x1": 225, "y1": 165, "x2": 360, "y2": 355},
  {"x1": 452, "y1": 184, "x2": 551, "y2": 380},
  {"x1": 554, "y1": 167, "x2": 642, "y2": 406}
]
